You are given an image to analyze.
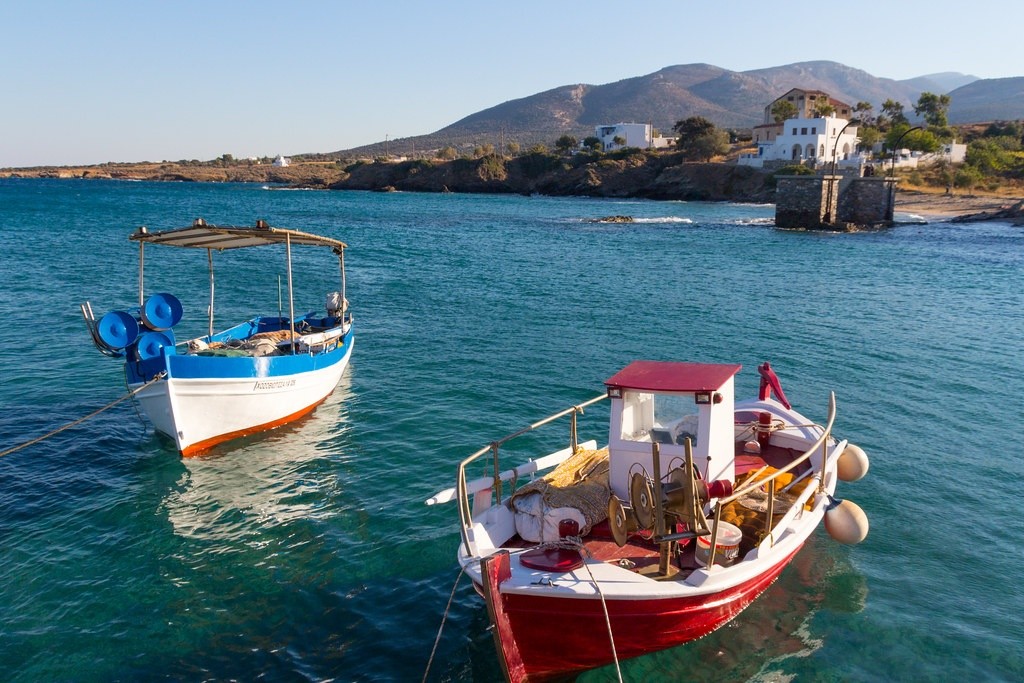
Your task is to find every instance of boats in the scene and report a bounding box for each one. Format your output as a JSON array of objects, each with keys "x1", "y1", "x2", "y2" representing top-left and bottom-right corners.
[
  {"x1": 457, "y1": 359, "x2": 849, "y2": 683},
  {"x1": 123, "y1": 314, "x2": 354, "y2": 459}
]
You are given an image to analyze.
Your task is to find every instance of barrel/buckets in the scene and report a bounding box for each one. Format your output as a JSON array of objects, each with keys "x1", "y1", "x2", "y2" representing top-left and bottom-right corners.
[{"x1": 694, "y1": 519, "x2": 742, "y2": 568}]
